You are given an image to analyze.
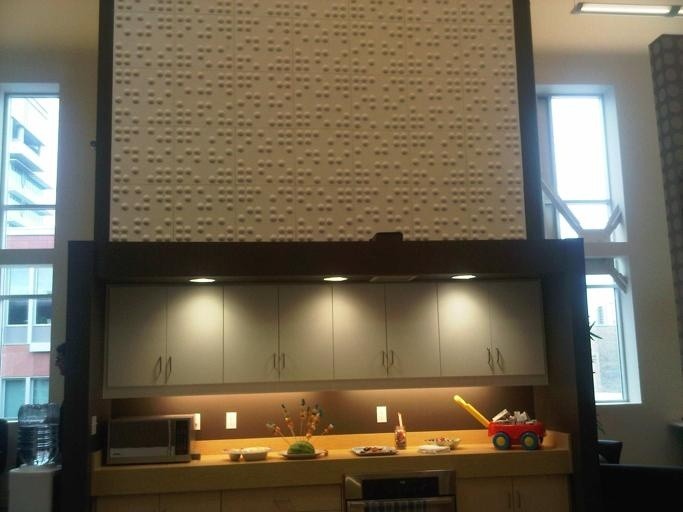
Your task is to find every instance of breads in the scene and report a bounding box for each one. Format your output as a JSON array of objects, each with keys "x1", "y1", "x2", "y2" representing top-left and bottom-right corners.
[{"x1": 419, "y1": 445, "x2": 449, "y2": 454}]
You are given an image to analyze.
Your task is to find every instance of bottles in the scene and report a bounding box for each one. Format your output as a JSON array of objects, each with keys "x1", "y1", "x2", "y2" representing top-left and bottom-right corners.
[{"x1": 394, "y1": 425, "x2": 406, "y2": 450}]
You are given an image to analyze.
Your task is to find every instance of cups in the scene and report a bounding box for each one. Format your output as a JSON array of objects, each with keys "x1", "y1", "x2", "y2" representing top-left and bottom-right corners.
[{"x1": 229, "y1": 452, "x2": 241, "y2": 461}]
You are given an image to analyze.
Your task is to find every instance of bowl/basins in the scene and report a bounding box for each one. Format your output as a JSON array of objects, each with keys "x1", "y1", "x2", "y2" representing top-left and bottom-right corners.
[
  {"x1": 424, "y1": 437, "x2": 460, "y2": 450},
  {"x1": 241, "y1": 448, "x2": 268, "y2": 460}
]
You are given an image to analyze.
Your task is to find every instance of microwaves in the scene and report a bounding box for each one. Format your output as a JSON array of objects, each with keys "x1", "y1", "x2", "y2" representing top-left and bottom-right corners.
[{"x1": 105, "y1": 413, "x2": 194, "y2": 467}]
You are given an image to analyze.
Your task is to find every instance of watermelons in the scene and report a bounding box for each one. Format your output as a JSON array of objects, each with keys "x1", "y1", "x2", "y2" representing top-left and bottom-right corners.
[{"x1": 287, "y1": 441, "x2": 315, "y2": 455}]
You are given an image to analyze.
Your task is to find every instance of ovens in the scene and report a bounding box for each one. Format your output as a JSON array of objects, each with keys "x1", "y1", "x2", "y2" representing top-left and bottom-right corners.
[{"x1": 339, "y1": 468, "x2": 457, "y2": 511}]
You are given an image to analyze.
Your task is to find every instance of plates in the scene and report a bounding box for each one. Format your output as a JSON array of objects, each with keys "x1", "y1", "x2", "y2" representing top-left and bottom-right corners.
[
  {"x1": 350, "y1": 446, "x2": 396, "y2": 456},
  {"x1": 282, "y1": 450, "x2": 322, "y2": 459}
]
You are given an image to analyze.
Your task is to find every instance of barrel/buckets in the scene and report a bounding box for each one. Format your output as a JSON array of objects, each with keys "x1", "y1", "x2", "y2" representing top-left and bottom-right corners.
[{"x1": 17, "y1": 404, "x2": 59, "y2": 465}]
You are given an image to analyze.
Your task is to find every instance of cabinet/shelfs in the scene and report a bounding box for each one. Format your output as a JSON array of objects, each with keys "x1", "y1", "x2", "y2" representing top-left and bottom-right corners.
[
  {"x1": 331, "y1": 279, "x2": 440, "y2": 393},
  {"x1": 221, "y1": 482, "x2": 344, "y2": 512},
  {"x1": 223, "y1": 279, "x2": 333, "y2": 392},
  {"x1": 438, "y1": 275, "x2": 550, "y2": 387},
  {"x1": 455, "y1": 474, "x2": 573, "y2": 512},
  {"x1": 104, "y1": 284, "x2": 224, "y2": 398},
  {"x1": 94, "y1": 490, "x2": 221, "y2": 512}
]
